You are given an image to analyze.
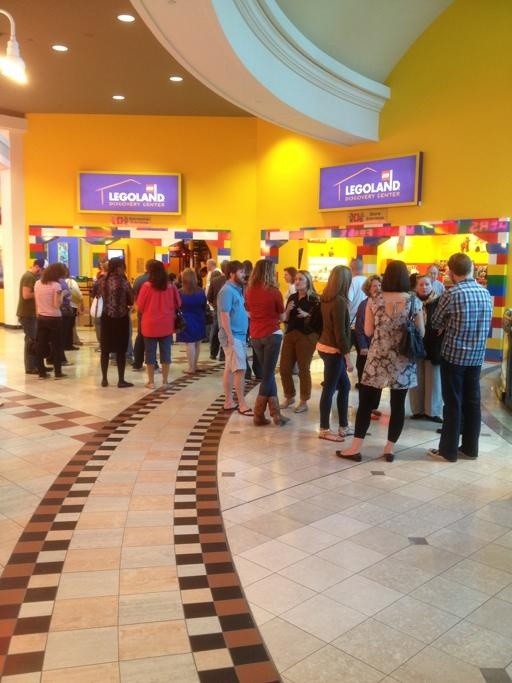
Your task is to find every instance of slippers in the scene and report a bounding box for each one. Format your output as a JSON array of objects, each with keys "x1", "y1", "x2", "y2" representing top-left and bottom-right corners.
[
  {"x1": 239, "y1": 408, "x2": 255, "y2": 416},
  {"x1": 222, "y1": 403, "x2": 240, "y2": 411}
]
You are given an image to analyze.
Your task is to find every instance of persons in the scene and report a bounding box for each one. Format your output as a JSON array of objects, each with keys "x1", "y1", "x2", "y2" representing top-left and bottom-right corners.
[
  {"x1": 17, "y1": 257, "x2": 54, "y2": 374},
  {"x1": 429, "y1": 253, "x2": 492, "y2": 460},
  {"x1": 205, "y1": 259, "x2": 229, "y2": 361},
  {"x1": 280, "y1": 270, "x2": 323, "y2": 411},
  {"x1": 320, "y1": 259, "x2": 368, "y2": 390},
  {"x1": 91, "y1": 257, "x2": 135, "y2": 388},
  {"x1": 245, "y1": 260, "x2": 290, "y2": 425},
  {"x1": 217, "y1": 260, "x2": 255, "y2": 416},
  {"x1": 408, "y1": 275, "x2": 444, "y2": 422},
  {"x1": 34, "y1": 263, "x2": 69, "y2": 380},
  {"x1": 427, "y1": 265, "x2": 445, "y2": 296},
  {"x1": 335, "y1": 259, "x2": 425, "y2": 461},
  {"x1": 285, "y1": 267, "x2": 298, "y2": 374},
  {"x1": 355, "y1": 275, "x2": 382, "y2": 420},
  {"x1": 136, "y1": 261, "x2": 180, "y2": 388},
  {"x1": 133, "y1": 259, "x2": 160, "y2": 371},
  {"x1": 47, "y1": 267, "x2": 83, "y2": 365},
  {"x1": 177, "y1": 268, "x2": 206, "y2": 373},
  {"x1": 317, "y1": 265, "x2": 361, "y2": 442}
]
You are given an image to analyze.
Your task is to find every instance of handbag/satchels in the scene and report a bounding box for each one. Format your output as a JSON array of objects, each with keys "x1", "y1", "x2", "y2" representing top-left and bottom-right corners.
[
  {"x1": 90, "y1": 296, "x2": 103, "y2": 318},
  {"x1": 303, "y1": 302, "x2": 322, "y2": 335},
  {"x1": 399, "y1": 319, "x2": 427, "y2": 360},
  {"x1": 203, "y1": 304, "x2": 213, "y2": 325},
  {"x1": 175, "y1": 311, "x2": 187, "y2": 335},
  {"x1": 61, "y1": 306, "x2": 75, "y2": 318}
]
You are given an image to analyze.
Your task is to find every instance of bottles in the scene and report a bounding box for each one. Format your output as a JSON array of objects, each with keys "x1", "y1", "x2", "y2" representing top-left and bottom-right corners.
[
  {"x1": 440, "y1": 260, "x2": 487, "y2": 288},
  {"x1": 71, "y1": 275, "x2": 88, "y2": 281},
  {"x1": 328, "y1": 247, "x2": 334, "y2": 257}
]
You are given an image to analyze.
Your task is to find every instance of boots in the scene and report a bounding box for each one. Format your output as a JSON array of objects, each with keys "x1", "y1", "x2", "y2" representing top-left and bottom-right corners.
[
  {"x1": 268, "y1": 396, "x2": 289, "y2": 426},
  {"x1": 253, "y1": 395, "x2": 271, "y2": 426}
]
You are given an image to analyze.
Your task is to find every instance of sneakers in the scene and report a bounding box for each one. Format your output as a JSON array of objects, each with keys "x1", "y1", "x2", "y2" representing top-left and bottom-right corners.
[
  {"x1": 318, "y1": 410, "x2": 476, "y2": 462},
  {"x1": 25, "y1": 341, "x2": 83, "y2": 380},
  {"x1": 95, "y1": 347, "x2": 206, "y2": 390},
  {"x1": 279, "y1": 399, "x2": 294, "y2": 408},
  {"x1": 294, "y1": 404, "x2": 308, "y2": 412}
]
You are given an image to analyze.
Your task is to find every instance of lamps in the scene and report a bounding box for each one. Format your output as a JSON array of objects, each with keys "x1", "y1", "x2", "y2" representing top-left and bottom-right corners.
[{"x1": 0, "y1": 10, "x2": 27, "y2": 82}]
